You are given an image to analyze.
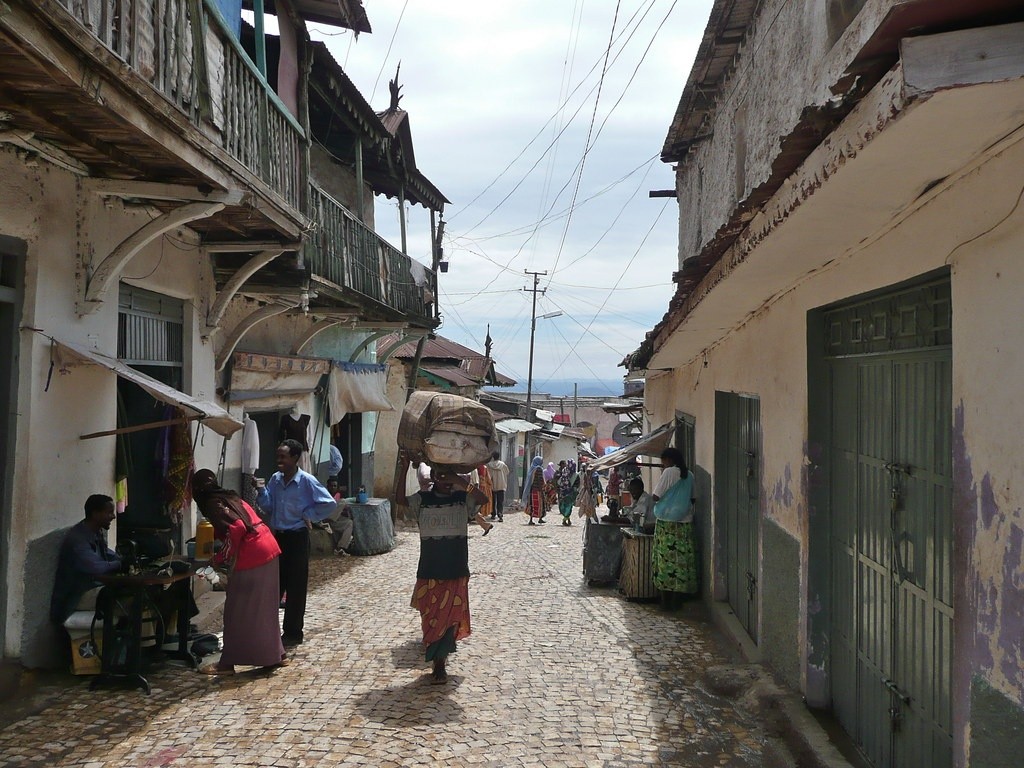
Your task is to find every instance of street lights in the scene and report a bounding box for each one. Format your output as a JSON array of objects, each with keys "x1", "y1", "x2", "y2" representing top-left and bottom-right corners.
[{"x1": 521, "y1": 307, "x2": 564, "y2": 500}]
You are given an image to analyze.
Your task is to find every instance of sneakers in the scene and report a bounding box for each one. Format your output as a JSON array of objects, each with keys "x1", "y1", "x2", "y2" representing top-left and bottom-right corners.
[
  {"x1": 316, "y1": 520, "x2": 333, "y2": 535},
  {"x1": 334, "y1": 548, "x2": 351, "y2": 557}
]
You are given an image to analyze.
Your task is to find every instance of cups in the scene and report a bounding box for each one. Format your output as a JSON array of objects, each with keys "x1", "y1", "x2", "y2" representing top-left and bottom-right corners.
[
  {"x1": 188, "y1": 542, "x2": 197, "y2": 558},
  {"x1": 356, "y1": 493, "x2": 368, "y2": 502}
]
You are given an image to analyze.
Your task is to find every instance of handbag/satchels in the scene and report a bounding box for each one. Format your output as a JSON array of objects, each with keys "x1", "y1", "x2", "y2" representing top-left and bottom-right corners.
[{"x1": 653, "y1": 471, "x2": 693, "y2": 523}]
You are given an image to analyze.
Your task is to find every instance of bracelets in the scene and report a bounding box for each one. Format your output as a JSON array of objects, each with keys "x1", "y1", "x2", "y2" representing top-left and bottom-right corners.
[{"x1": 466, "y1": 483, "x2": 474, "y2": 494}]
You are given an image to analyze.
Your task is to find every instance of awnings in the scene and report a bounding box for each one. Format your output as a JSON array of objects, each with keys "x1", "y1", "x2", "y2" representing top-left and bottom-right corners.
[
  {"x1": 586, "y1": 420, "x2": 676, "y2": 472},
  {"x1": 495, "y1": 418, "x2": 542, "y2": 433},
  {"x1": 44, "y1": 335, "x2": 244, "y2": 439}
]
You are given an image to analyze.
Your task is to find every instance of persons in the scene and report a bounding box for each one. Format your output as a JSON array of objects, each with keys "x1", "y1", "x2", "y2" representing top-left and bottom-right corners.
[
  {"x1": 249, "y1": 439, "x2": 338, "y2": 647},
  {"x1": 326, "y1": 478, "x2": 353, "y2": 555},
  {"x1": 487, "y1": 451, "x2": 509, "y2": 522},
  {"x1": 624, "y1": 479, "x2": 657, "y2": 523},
  {"x1": 51, "y1": 492, "x2": 140, "y2": 677},
  {"x1": 467, "y1": 467, "x2": 494, "y2": 537},
  {"x1": 395, "y1": 447, "x2": 489, "y2": 685},
  {"x1": 521, "y1": 455, "x2": 623, "y2": 525},
  {"x1": 653, "y1": 448, "x2": 699, "y2": 606},
  {"x1": 190, "y1": 469, "x2": 288, "y2": 675}
]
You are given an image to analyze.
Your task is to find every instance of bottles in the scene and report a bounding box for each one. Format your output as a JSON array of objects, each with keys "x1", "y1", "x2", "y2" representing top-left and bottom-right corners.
[{"x1": 195, "y1": 516, "x2": 215, "y2": 562}]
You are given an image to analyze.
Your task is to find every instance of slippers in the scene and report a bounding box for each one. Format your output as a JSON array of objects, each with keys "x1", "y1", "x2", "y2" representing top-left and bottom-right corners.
[
  {"x1": 198, "y1": 664, "x2": 236, "y2": 675},
  {"x1": 281, "y1": 658, "x2": 289, "y2": 665}
]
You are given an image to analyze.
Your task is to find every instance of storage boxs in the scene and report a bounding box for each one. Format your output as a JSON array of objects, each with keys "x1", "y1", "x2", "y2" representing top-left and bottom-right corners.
[{"x1": 72, "y1": 629, "x2": 104, "y2": 675}]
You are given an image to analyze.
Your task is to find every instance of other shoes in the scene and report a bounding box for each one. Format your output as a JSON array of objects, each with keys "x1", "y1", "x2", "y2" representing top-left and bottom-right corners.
[
  {"x1": 538, "y1": 520, "x2": 546, "y2": 523},
  {"x1": 281, "y1": 635, "x2": 303, "y2": 646},
  {"x1": 419, "y1": 671, "x2": 448, "y2": 685},
  {"x1": 482, "y1": 524, "x2": 494, "y2": 536},
  {"x1": 529, "y1": 522, "x2": 535, "y2": 524}
]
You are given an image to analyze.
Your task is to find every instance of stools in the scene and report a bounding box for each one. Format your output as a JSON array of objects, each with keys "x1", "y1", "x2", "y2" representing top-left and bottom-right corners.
[{"x1": 311, "y1": 529, "x2": 335, "y2": 555}]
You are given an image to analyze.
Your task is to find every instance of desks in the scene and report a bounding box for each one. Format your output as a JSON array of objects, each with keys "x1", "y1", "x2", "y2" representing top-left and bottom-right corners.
[{"x1": 351, "y1": 498, "x2": 397, "y2": 555}]
